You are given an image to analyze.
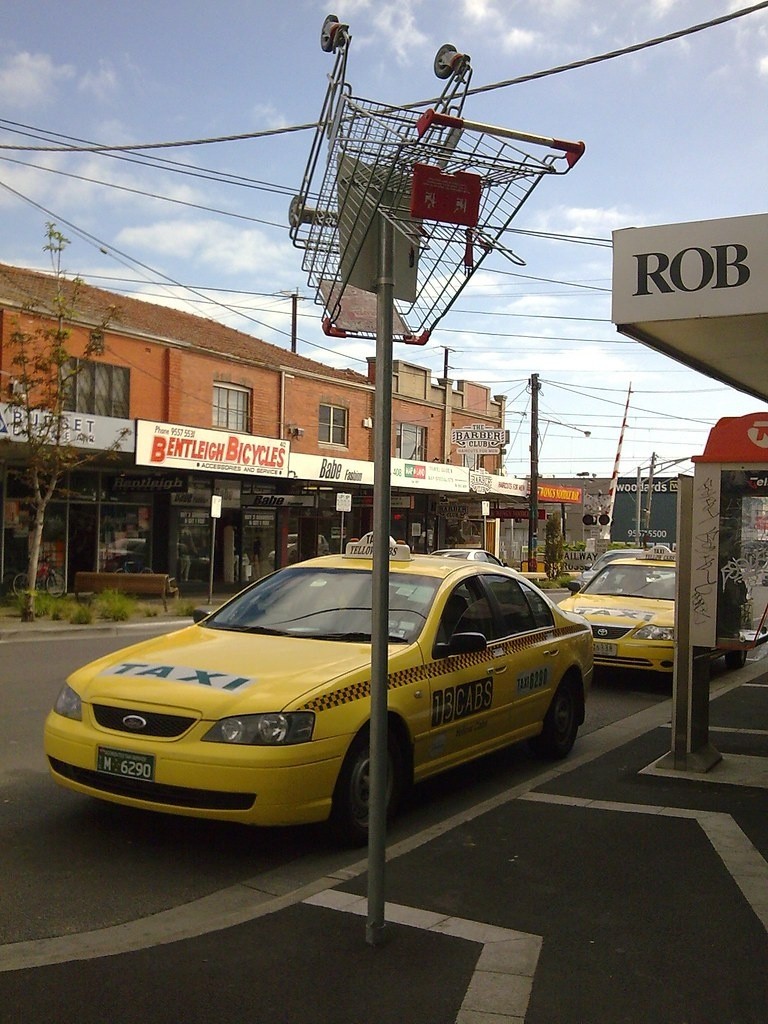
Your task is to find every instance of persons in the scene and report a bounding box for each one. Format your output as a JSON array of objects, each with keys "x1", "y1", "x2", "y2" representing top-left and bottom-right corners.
[
  {"x1": 178, "y1": 538, "x2": 191, "y2": 582},
  {"x1": 252, "y1": 537, "x2": 262, "y2": 580}
]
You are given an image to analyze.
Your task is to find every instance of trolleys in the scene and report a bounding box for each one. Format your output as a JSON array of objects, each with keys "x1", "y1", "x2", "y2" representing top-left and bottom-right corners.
[{"x1": 286, "y1": 12, "x2": 585, "y2": 346}]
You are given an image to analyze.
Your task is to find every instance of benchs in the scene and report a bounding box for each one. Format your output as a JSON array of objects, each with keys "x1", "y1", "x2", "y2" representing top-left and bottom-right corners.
[{"x1": 74, "y1": 572, "x2": 180, "y2": 612}]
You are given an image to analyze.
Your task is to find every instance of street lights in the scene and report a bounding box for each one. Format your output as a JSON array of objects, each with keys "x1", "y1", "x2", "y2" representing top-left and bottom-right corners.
[{"x1": 527, "y1": 415, "x2": 591, "y2": 572}]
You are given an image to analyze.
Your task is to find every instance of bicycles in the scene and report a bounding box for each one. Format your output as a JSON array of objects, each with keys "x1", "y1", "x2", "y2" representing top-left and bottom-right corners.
[
  {"x1": 112, "y1": 558, "x2": 155, "y2": 575},
  {"x1": 13, "y1": 556, "x2": 66, "y2": 599}
]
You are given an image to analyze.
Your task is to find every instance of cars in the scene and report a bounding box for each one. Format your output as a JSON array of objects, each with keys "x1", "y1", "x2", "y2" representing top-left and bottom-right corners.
[
  {"x1": 42, "y1": 528, "x2": 597, "y2": 854},
  {"x1": 570, "y1": 549, "x2": 646, "y2": 597},
  {"x1": 100, "y1": 534, "x2": 210, "y2": 583},
  {"x1": 555, "y1": 541, "x2": 748, "y2": 695},
  {"x1": 428, "y1": 547, "x2": 533, "y2": 595}
]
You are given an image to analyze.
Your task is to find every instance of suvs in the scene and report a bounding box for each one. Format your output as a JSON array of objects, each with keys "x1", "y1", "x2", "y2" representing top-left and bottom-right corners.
[{"x1": 269, "y1": 532, "x2": 331, "y2": 570}]
